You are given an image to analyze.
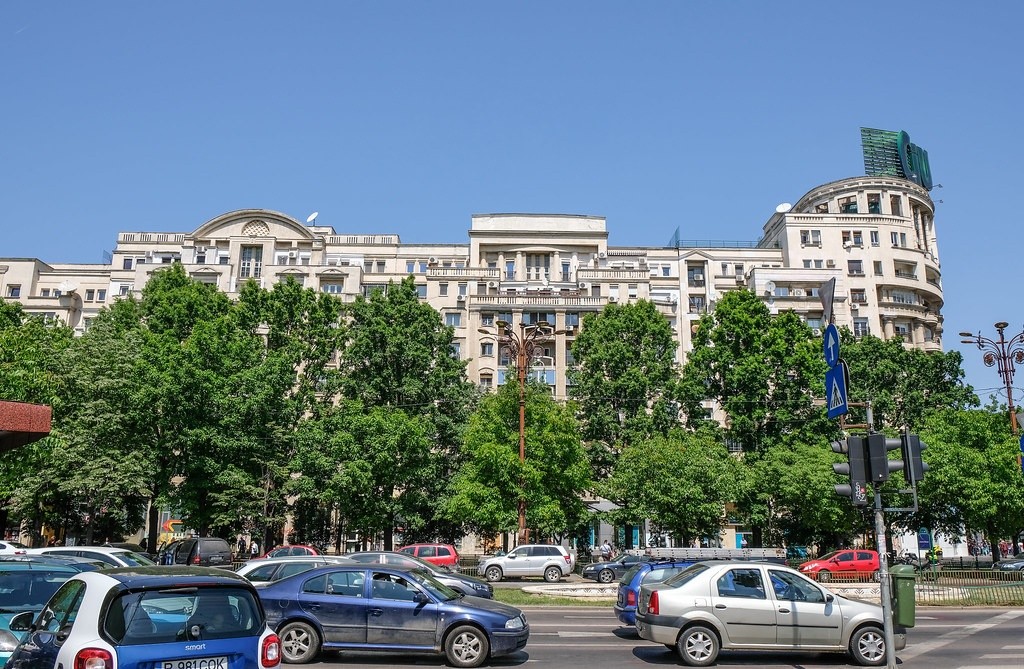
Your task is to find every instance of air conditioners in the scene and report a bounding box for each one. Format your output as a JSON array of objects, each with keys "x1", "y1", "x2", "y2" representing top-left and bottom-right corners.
[
  {"x1": 488, "y1": 281, "x2": 499, "y2": 289},
  {"x1": 429, "y1": 257, "x2": 438, "y2": 264},
  {"x1": 735, "y1": 275, "x2": 745, "y2": 282},
  {"x1": 578, "y1": 282, "x2": 587, "y2": 289},
  {"x1": 598, "y1": 251, "x2": 607, "y2": 258},
  {"x1": 694, "y1": 274, "x2": 704, "y2": 281},
  {"x1": 850, "y1": 303, "x2": 859, "y2": 310},
  {"x1": 289, "y1": 251, "x2": 297, "y2": 259},
  {"x1": 793, "y1": 288, "x2": 804, "y2": 296},
  {"x1": 827, "y1": 259, "x2": 836, "y2": 266},
  {"x1": 608, "y1": 296, "x2": 618, "y2": 303},
  {"x1": 638, "y1": 257, "x2": 647, "y2": 265},
  {"x1": 197, "y1": 246, "x2": 206, "y2": 253},
  {"x1": 456, "y1": 295, "x2": 466, "y2": 302}
]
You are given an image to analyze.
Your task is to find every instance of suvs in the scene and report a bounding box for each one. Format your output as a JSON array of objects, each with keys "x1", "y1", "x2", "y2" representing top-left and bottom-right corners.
[
  {"x1": 369, "y1": 543, "x2": 461, "y2": 574},
  {"x1": 477, "y1": 544, "x2": 575, "y2": 583},
  {"x1": 152, "y1": 537, "x2": 235, "y2": 572}
]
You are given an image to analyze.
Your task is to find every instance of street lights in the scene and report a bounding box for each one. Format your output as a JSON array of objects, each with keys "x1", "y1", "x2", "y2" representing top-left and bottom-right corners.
[
  {"x1": 476, "y1": 320, "x2": 573, "y2": 545},
  {"x1": 959, "y1": 321, "x2": 1024, "y2": 477}
]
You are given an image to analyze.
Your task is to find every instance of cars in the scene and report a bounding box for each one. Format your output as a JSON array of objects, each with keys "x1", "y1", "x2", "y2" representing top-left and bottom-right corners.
[
  {"x1": 0, "y1": 539, "x2": 240, "y2": 669},
  {"x1": 990, "y1": 552, "x2": 1024, "y2": 582},
  {"x1": 254, "y1": 564, "x2": 530, "y2": 668},
  {"x1": 581, "y1": 553, "x2": 650, "y2": 584},
  {"x1": 3, "y1": 566, "x2": 282, "y2": 669},
  {"x1": 797, "y1": 549, "x2": 880, "y2": 583},
  {"x1": 233, "y1": 554, "x2": 420, "y2": 592},
  {"x1": 635, "y1": 560, "x2": 906, "y2": 667},
  {"x1": 250, "y1": 544, "x2": 325, "y2": 562},
  {"x1": 344, "y1": 550, "x2": 494, "y2": 599},
  {"x1": 613, "y1": 557, "x2": 790, "y2": 627}
]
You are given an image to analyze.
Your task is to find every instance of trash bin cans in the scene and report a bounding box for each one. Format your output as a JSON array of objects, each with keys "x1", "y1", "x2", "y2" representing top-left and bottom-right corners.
[{"x1": 888, "y1": 564, "x2": 918, "y2": 627}]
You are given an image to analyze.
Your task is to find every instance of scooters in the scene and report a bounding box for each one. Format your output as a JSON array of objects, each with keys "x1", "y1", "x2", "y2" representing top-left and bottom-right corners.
[{"x1": 894, "y1": 548, "x2": 943, "y2": 581}]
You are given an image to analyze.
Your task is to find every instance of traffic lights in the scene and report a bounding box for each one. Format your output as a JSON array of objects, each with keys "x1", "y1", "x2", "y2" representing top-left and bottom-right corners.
[
  {"x1": 862, "y1": 435, "x2": 904, "y2": 482},
  {"x1": 830, "y1": 435, "x2": 868, "y2": 506},
  {"x1": 900, "y1": 433, "x2": 931, "y2": 482}
]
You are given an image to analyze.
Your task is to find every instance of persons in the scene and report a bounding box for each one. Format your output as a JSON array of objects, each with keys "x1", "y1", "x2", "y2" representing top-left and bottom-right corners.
[
  {"x1": 599, "y1": 540, "x2": 616, "y2": 561},
  {"x1": 740, "y1": 537, "x2": 747, "y2": 548},
  {"x1": 251, "y1": 541, "x2": 259, "y2": 558},
  {"x1": 238, "y1": 537, "x2": 246, "y2": 553},
  {"x1": 139, "y1": 538, "x2": 147, "y2": 550},
  {"x1": 372, "y1": 575, "x2": 388, "y2": 597},
  {"x1": 1000, "y1": 541, "x2": 1008, "y2": 558},
  {"x1": 47, "y1": 536, "x2": 55, "y2": 546},
  {"x1": 327, "y1": 579, "x2": 333, "y2": 593}
]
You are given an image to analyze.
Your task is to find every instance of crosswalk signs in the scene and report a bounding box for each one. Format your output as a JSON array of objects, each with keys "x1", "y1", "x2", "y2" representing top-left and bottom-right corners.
[{"x1": 824, "y1": 363, "x2": 849, "y2": 419}]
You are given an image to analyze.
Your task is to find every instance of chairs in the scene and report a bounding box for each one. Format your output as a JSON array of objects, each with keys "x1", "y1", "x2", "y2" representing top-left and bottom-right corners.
[{"x1": 177, "y1": 594, "x2": 242, "y2": 637}]
